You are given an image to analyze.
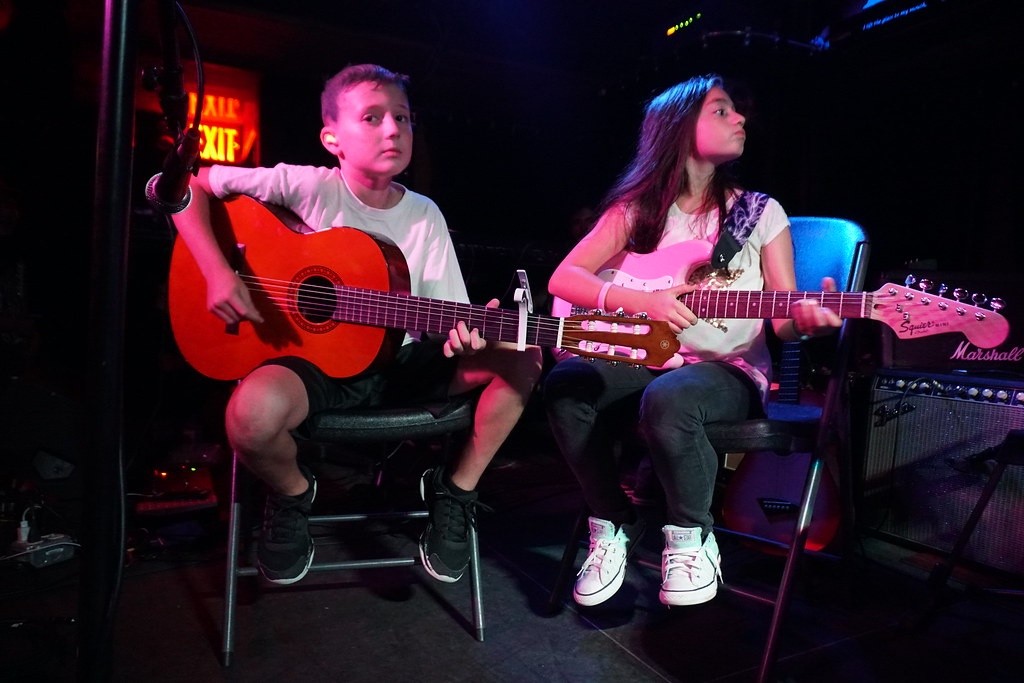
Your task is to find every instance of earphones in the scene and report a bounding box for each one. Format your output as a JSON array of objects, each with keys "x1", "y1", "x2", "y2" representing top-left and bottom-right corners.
[{"x1": 325, "y1": 134, "x2": 336, "y2": 143}]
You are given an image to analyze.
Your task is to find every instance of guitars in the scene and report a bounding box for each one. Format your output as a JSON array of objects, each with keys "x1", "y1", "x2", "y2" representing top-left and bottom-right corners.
[
  {"x1": 548, "y1": 238, "x2": 1012, "y2": 371},
  {"x1": 167, "y1": 191, "x2": 682, "y2": 382},
  {"x1": 721, "y1": 339, "x2": 846, "y2": 560},
  {"x1": 0, "y1": 199, "x2": 45, "y2": 389}
]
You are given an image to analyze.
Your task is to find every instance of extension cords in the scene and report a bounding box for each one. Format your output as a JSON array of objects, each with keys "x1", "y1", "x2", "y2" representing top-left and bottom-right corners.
[{"x1": 11, "y1": 531, "x2": 75, "y2": 568}]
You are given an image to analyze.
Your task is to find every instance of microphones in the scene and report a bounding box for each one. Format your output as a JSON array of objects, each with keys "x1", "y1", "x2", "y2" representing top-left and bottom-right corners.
[
  {"x1": 145, "y1": 125, "x2": 204, "y2": 214},
  {"x1": 983, "y1": 429, "x2": 1024, "y2": 474}
]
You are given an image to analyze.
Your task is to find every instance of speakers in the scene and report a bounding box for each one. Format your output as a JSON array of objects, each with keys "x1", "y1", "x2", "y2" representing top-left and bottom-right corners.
[{"x1": 860, "y1": 366, "x2": 1024, "y2": 579}]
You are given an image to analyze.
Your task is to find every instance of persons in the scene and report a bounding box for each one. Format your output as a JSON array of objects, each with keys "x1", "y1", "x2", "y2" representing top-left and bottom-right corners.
[
  {"x1": 170, "y1": 64, "x2": 543, "y2": 584},
  {"x1": 545, "y1": 74, "x2": 843, "y2": 609}
]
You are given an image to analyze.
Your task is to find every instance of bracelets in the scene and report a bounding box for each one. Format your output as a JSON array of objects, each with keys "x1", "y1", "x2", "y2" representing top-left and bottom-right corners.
[
  {"x1": 792, "y1": 320, "x2": 805, "y2": 337},
  {"x1": 598, "y1": 281, "x2": 616, "y2": 313}
]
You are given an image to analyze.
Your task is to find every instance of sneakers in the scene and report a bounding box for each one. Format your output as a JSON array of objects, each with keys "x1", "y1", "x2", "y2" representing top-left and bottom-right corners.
[
  {"x1": 419, "y1": 465, "x2": 493, "y2": 583},
  {"x1": 659, "y1": 525, "x2": 724, "y2": 606},
  {"x1": 573, "y1": 508, "x2": 647, "y2": 606},
  {"x1": 257, "y1": 464, "x2": 317, "y2": 585}
]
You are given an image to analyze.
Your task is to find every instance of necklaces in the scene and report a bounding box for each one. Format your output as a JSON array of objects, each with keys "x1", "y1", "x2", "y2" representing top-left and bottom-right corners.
[{"x1": 340, "y1": 169, "x2": 388, "y2": 210}]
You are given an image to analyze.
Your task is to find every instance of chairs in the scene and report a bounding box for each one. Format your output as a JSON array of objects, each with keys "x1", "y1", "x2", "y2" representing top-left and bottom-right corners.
[
  {"x1": 220, "y1": 382, "x2": 485, "y2": 667},
  {"x1": 549, "y1": 216, "x2": 867, "y2": 683}
]
